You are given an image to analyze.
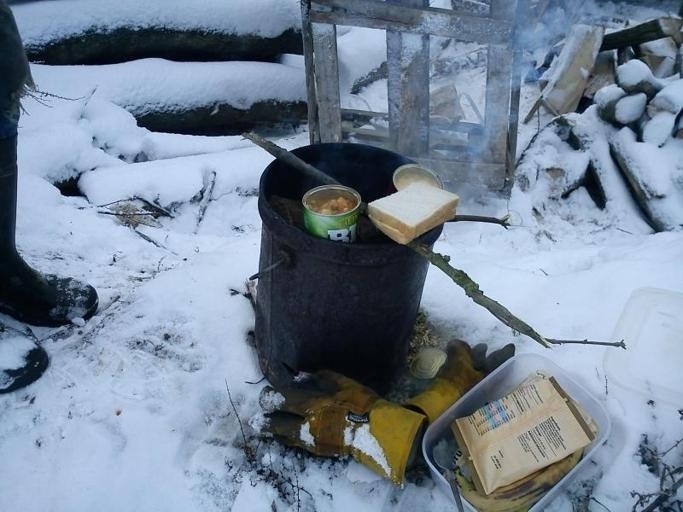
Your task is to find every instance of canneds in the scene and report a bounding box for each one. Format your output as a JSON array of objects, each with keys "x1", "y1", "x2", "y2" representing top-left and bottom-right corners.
[
  {"x1": 302, "y1": 185, "x2": 361, "y2": 242},
  {"x1": 391, "y1": 164, "x2": 444, "y2": 197}
]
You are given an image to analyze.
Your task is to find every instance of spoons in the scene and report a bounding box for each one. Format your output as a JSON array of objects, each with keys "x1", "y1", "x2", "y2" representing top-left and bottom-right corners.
[{"x1": 432, "y1": 438, "x2": 465, "y2": 512}]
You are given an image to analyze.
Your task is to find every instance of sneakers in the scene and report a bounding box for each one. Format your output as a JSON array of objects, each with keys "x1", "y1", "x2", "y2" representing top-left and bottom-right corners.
[{"x1": 0, "y1": 323, "x2": 49, "y2": 395}]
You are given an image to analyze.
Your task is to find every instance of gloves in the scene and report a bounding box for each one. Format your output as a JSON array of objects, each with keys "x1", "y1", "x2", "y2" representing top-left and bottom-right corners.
[
  {"x1": 245, "y1": 353, "x2": 431, "y2": 491},
  {"x1": 401, "y1": 338, "x2": 516, "y2": 426}
]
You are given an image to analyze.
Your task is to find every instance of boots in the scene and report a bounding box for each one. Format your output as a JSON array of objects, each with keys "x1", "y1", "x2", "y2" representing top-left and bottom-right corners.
[{"x1": 0, "y1": 130, "x2": 100, "y2": 328}]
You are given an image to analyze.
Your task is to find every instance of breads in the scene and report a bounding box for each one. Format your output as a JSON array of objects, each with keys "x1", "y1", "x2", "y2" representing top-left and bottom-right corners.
[{"x1": 368, "y1": 180, "x2": 461, "y2": 244}]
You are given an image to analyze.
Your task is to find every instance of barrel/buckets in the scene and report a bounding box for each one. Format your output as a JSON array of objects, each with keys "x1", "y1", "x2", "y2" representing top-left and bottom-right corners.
[{"x1": 248, "y1": 141, "x2": 446, "y2": 403}]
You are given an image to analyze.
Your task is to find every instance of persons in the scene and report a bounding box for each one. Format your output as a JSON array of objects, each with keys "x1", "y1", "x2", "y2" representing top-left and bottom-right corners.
[{"x1": 0, "y1": 2, "x2": 99, "y2": 395}]
